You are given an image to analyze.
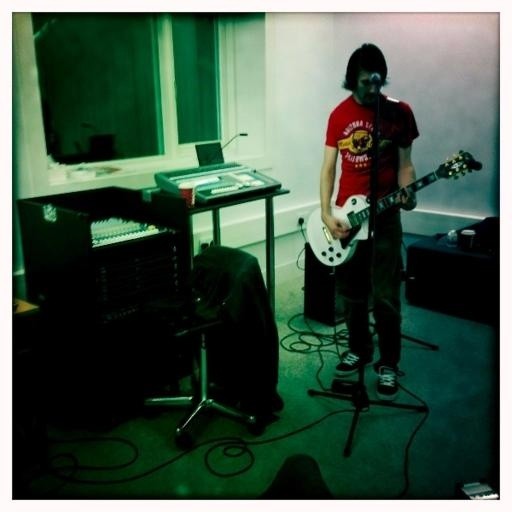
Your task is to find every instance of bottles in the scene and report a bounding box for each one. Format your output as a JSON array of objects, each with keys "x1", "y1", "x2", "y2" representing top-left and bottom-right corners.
[{"x1": 446, "y1": 229, "x2": 458, "y2": 248}]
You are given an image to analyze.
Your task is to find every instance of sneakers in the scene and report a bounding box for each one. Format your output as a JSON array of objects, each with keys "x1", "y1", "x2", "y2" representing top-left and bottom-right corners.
[
  {"x1": 376, "y1": 364, "x2": 400, "y2": 404},
  {"x1": 334, "y1": 349, "x2": 375, "y2": 376}
]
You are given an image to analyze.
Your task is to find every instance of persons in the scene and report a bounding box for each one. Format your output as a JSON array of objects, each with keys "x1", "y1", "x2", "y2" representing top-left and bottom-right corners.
[{"x1": 319, "y1": 42, "x2": 421, "y2": 402}]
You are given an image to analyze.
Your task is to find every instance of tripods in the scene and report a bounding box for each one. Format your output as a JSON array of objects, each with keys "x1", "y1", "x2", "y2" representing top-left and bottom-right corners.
[{"x1": 308, "y1": 96, "x2": 429, "y2": 458}]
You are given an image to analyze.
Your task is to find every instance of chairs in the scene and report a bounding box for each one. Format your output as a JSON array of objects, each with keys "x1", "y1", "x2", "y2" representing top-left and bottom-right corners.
[{"x1": 134, "y1": 243, "x2": 265, "y2": 450}]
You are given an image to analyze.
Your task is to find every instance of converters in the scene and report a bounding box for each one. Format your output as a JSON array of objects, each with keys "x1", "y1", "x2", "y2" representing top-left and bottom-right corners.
[{"x1": 332, "y1": 378, "x2": 356, "y2": 393}]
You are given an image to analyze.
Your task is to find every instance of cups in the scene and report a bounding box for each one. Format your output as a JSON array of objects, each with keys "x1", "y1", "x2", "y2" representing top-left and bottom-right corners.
[{"x1": 459, "y1": 229, "x2": 477, "y2": 250}]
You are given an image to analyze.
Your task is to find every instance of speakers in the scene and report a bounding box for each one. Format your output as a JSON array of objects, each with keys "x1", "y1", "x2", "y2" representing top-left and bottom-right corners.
[
  {"x1": 304, "y1": 242, "x2": 375, "y2": 326},
  {"x1": 405, "y1": 233, "x2": 500, "y2": 325},
  {"x1": 193, "y1": 245, "x2": 279, "y2": 416}
]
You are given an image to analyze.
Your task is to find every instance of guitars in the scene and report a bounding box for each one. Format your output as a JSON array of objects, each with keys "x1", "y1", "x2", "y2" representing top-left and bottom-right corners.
[{"x1": 305, "y1": 150, "x2": 482, "y2": 267}]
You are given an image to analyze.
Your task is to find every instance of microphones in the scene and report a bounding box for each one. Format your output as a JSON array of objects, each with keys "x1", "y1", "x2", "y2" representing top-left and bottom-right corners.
[
  {"x1": 206, "y1": 134, "x2": 248, "y2": 164},
  {"x1": 367, "y1": 72, "x2": 381, "y2": 96}
]
